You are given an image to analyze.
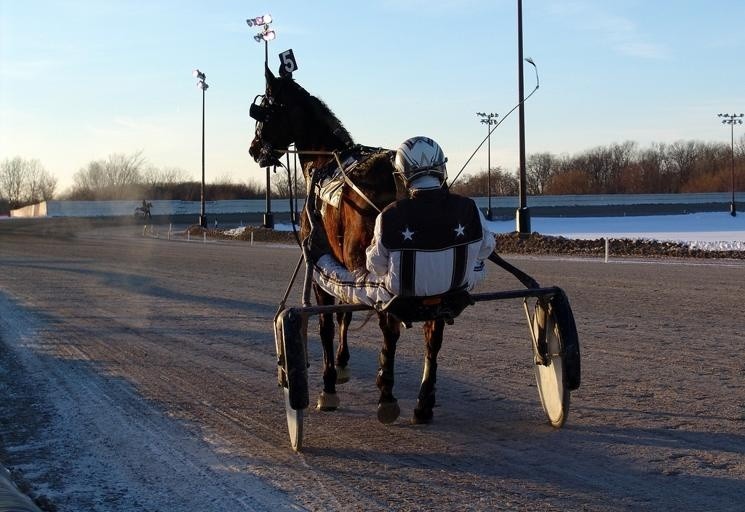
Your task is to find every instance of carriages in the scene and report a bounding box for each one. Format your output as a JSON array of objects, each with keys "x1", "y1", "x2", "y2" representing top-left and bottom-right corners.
[
  {"x1": 248, "y1": 59, "x2": 581, "y2": 452},
  {"x1": 133, "y1": 199, "x2": 153, "y2": 220}
]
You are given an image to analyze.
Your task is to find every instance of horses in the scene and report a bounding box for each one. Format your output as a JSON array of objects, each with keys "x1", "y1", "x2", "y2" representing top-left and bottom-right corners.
[
  {"x1": 135, "y1": 202, "x2": 153, "y2": 220},
  {"x1": 249, "y1": 63, "x2": 445, "y2": 425}
]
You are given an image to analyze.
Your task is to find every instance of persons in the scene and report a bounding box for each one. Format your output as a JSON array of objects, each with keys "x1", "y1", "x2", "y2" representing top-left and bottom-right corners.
[
  {"x1": 301, "y1": 136, "x2": 496, "y2": 311},
  {"x1": 143, "y1": 199, "x2": 146, "y2": 206}
]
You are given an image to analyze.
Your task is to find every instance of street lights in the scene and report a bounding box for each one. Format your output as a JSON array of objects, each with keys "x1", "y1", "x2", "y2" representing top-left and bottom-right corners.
[
  {"x1": 476, "y1": 111, "x2": 500, "y2": 221},
  {"x1": 246, "y1": 13, "x2": 276, "y2": 228},
  {"x1": 717, "y1": 112, "x2": 745, "y2": 217},
  {"x1": 192, "y1": 68, "x2": 210, "y2": 227}
]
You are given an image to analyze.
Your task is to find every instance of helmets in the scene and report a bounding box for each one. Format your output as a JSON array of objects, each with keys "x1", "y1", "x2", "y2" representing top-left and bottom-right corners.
[{"x1": 393, "y1": 135, "x2": 449, "y2": 192}]
